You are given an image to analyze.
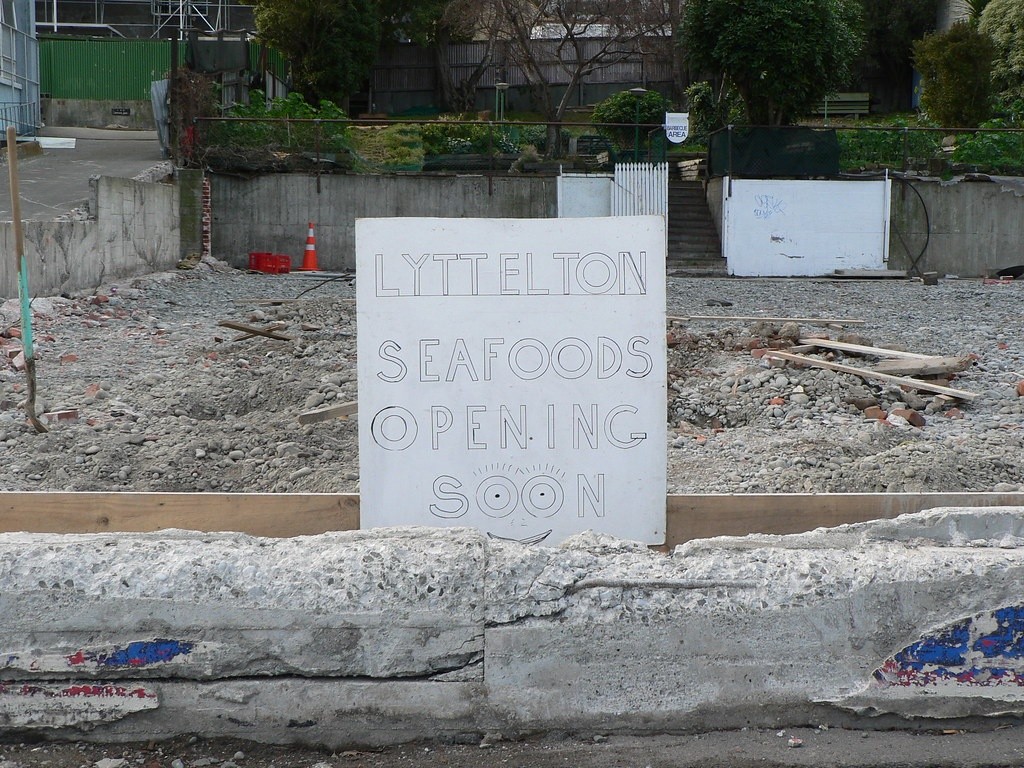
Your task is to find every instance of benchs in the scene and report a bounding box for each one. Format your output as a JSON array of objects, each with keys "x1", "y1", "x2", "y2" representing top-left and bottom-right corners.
[
  {"x1": 573, "y1": 135, "x2": 614, "y2": 164},
  {"x1": 809, "y1": 92, "x2": 870, "y2": 118}
]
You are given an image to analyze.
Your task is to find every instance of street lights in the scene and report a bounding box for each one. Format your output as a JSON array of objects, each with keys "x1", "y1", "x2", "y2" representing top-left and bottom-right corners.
[
  {"x1": 494, "y1": 82, "x2": 511, "y2": 127},
  {"x1": 628, "y1": 87, "x2": 648, "y2": 163}
]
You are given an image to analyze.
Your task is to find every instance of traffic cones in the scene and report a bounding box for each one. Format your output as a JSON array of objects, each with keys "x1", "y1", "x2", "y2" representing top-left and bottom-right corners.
[{"x1": 296, "y1": 216, "x2": 322, "y2": 270}]
